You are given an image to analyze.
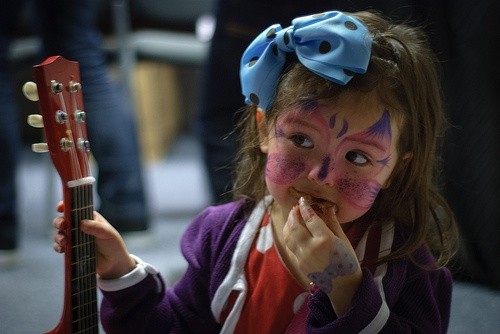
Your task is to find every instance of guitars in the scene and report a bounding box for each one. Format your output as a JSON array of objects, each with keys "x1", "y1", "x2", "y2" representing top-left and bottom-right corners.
[{"x1": 22, "y1": 55, "x2": 100, "y2": 334}]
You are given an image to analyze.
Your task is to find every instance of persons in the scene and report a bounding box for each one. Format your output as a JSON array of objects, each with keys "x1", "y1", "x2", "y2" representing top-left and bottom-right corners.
[
  {"x1": 54, "y1": 11, "x2": 460, "y2": 334},
  {"x1": 0, "y1": 0, "x2": 500, "y2": 291}
]
